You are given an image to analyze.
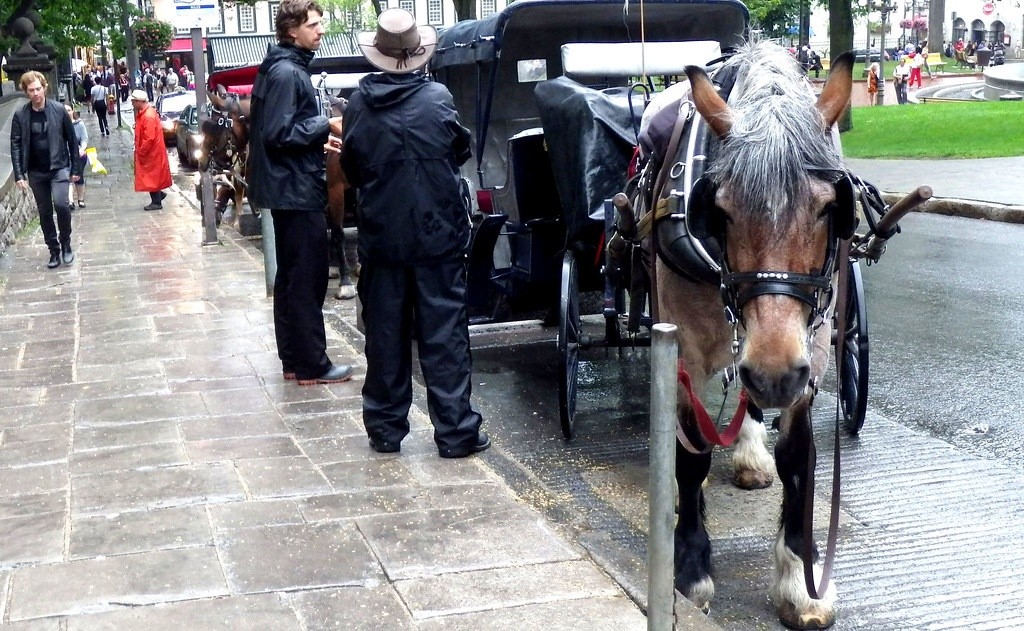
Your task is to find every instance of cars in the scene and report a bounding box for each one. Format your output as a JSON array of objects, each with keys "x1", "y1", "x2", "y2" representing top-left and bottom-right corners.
[
  {"x1": 855, "y1": 49, "x2": 890, "y2": 62},
  {"x1": 177, "y1": 104, "x2": 211, "y2": 170},
  {"x1": 155, "y1": 86, "x2": 210, "y2": 149}
]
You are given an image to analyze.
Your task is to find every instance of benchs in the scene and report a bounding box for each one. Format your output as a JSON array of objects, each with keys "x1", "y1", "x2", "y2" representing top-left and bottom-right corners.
[
  {"x1": 955, "y1": 49, "x2": 977, "y2": 69},
  {"x1": 559, "y1": 41, "x2": 723, "y2": 108},
  {"x1": 927, "y1": 52, "x2": 948, "y2": 72}
]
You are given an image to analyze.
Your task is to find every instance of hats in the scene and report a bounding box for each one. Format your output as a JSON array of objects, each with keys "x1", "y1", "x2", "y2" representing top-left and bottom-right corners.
[
  {"x1": 128, "y1": 90, "x2": 148, "y2": 101},
  {"x1": 356, "y1": 7, "x2": 437, "y2": 75}
]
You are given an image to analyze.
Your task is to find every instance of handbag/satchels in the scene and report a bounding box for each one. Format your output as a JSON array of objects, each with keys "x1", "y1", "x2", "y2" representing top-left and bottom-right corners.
[
  {"x1": 92, "y1": 103, "x2": 95, "y2": 114},
  {"x1": 923, "y1": 53, "x2": 927, "y2": 59}
]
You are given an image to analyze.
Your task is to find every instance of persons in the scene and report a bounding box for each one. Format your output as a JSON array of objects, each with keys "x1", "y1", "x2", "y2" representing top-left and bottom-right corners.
[
  {"x1": 90, "y1": 76, "x2": 110, "y2": 137},
  {"x1": 128, "y1": 89, "x2": 173, "y2": 211},
  {"x1": 246, "y1": 0, "x2": 342, "y2": 385},
  {"x1": 893, "y1": 58, "x2": 911, "y2": 104},
  {"x1": 134, "y1": 60, "x2": 195, "y2": 102},
  {"x1": 10, "y1": 71, "x2": 82, "y2": 268},
  {"x1": 339, "y1": 7, "x2": 493, "y2": 459},
  {"x1": 56, "y1": 81, "x2": 68, "y2": 102},
  {"x1": 943, "y1": 37, "x2": 1006, "y2": 58},
  {"x1": 73, "y1": 61, "x2": 130, "y2": 103},
  {"x1": 795, "y1": 43, "x2": 823, "y2": 78},
  {"x1": 61, "y1": 102, "x2": 89, "y2": 211},
  {"x1": 893, "y1": 40, "x2": 937, "y2": 89},
  {"x1": 1014, "y1": 38, "x2": 1022, "y2": 59},
  {"x1": 867, "y1": 62, "x2": 880, "y2": 106}
]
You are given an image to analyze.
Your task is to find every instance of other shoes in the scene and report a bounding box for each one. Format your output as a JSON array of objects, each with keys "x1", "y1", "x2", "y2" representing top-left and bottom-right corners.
[
  {"x1": 144, "y1": 203, "x2": 162, "y2": 210},
  {"x1": 283, "y1": 369, "x2": 296, "y2": 379},
  {"x1": 106, "y1": 129, "x2": 109, "y2": 135},
  {"x1": 62, "y1": 241, "x2": 74, "y2": 263},
  {"x1": 161, "y1": 192, "x2": 167, "y2": 200},
  {"x1": 931, "y1": 76, "x2": 937, "y2": 81},
  {"x1": 69, "y1": 202, "x2": 75, "y2": 209},
  {"x1": 298, "y1": 365, "x2": 353, "y2": 385},
  {"x1": 48, "y1": 254, "x2": 61, "y2": 267},
  {"x1": 78, "y1": 199, "x2": 86, "y2": 208}
]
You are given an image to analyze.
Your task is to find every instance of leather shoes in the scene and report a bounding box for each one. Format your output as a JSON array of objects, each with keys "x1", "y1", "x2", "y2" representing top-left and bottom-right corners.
[
  {"x1": 367, "y1": 439, "x2": 401, "y2": 451},
  {"x1": 471, "y1": 430, "x2": 491, "y2": 452}
]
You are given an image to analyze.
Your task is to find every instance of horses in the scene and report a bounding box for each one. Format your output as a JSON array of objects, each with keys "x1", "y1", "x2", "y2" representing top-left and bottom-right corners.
[
  {"x1": 202, "y1": 84, "x2": 254, "y2": 228},
  {"x1": 325, "y1": 98, "x2": 361, "y2": 301},
  {"x1": 626, "y1": 24, "x2": 856, "y2": 631}
]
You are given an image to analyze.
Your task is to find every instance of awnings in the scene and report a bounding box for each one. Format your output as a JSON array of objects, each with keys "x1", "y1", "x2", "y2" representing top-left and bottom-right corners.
[{"x1": 206, "y1": 33, "x2": 368, "y2": 74}]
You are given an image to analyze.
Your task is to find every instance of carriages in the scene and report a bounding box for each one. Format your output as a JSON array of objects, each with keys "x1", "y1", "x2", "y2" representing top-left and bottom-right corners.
[
  {"x1": 429, "y1": 0, "x2": 935, "y2": 631},
  {"x1": 199, "y1": 55, "x2": 383, "y2": 300}
]
